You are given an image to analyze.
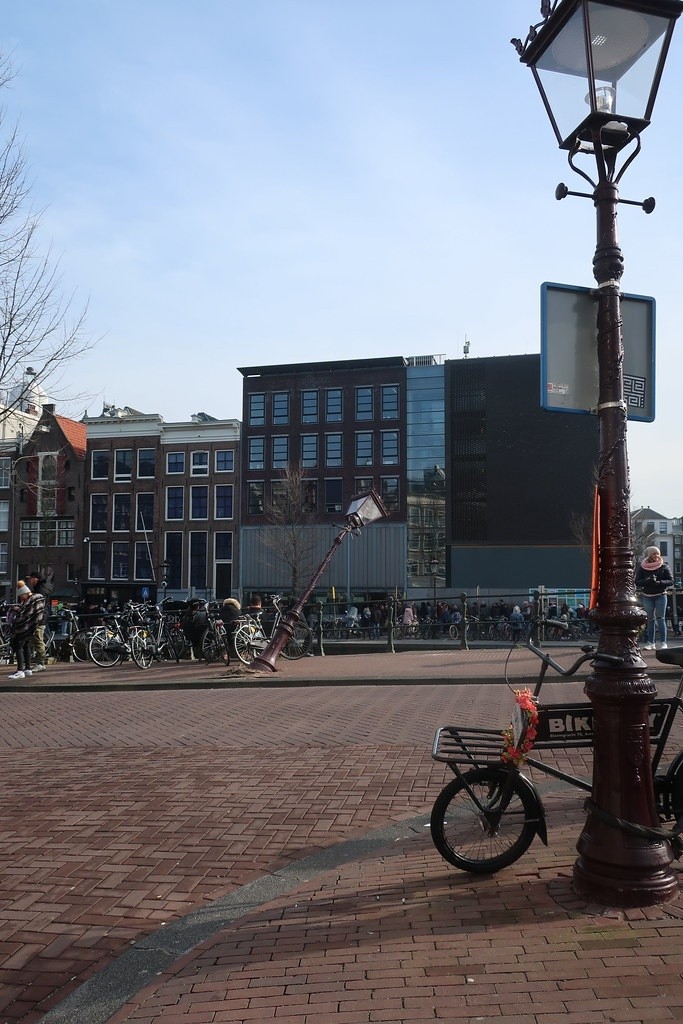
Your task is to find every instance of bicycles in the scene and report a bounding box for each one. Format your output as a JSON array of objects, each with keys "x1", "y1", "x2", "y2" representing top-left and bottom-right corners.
[
  {"x1": 432, "y1": 619, "x2": 682, "y2": 876},
  {"x1": 1, "y1": 596, "x2": 647, "y2": 670}
]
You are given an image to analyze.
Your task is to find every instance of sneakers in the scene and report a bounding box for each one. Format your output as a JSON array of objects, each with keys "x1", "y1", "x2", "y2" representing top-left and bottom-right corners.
[
  {"x1": 644, "y1": 641, "x2": 656, "y2": 650},
  {"x1": 24, "y1": 670, "x2": 32, "y2": 676},
  {"x1": 8, "y1": 671, "x2": 25, "y2": 679},
  {"x1": 660, "y1": 642, "x2": 667, "y2": 649},
  {"x1": 31, "y1": 664, "x2": 46, "y2": 672}
]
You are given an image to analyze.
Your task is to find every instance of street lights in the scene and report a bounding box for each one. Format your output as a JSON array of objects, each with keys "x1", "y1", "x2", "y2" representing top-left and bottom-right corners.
[
  {"x1": 247, "y1": 488, "x2": 389, "y2": 671},
  {"x1": 512, "y1": 1, "x2": 681, "y2": 906}
]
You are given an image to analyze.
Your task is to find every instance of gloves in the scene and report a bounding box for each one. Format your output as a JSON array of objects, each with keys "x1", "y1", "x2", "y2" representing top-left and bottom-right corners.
[
  {"x1": 649, "y1": 575, "x2": 657, "y2": 582},
  {"x1": 654, "y1": 581, "x2": 660, "y2": 585}
]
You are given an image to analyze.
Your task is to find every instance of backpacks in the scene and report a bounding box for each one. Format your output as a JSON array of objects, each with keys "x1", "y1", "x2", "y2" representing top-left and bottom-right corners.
[{"x1": 511, "y1": 613, "x2": 520, "y2": 626}]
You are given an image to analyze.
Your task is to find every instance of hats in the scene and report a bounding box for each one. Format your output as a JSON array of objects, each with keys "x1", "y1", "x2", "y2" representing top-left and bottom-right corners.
[
  {"x1": 17, "y1": 580, "x2": 31, "y2": 596},
  {"x1": 30, "y1": 571, "x2": 41, "y2": 579},
  {"x1": 644, "y1": 546, "x2": 661, "y2": 560}
]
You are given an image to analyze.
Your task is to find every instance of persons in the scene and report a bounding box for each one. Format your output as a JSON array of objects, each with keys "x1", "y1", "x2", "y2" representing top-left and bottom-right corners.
[
  {"x1": 7, "y1": 581, "x2": 45, "y2": 678},
  {"x1": 218, "y1": 582, "x2": 601, "y2": 641},
  {"x1": 636, "y1": 544, "x2": 676, "y2": 653},
  {"x1": 26, "y1": 571, "x2": 54, "y2": 672}
]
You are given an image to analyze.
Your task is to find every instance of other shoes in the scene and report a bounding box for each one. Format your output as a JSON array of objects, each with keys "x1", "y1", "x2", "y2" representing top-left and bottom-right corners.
[{"x1": 61, "y1": 633, "x2": 68, "y2": 636}]
